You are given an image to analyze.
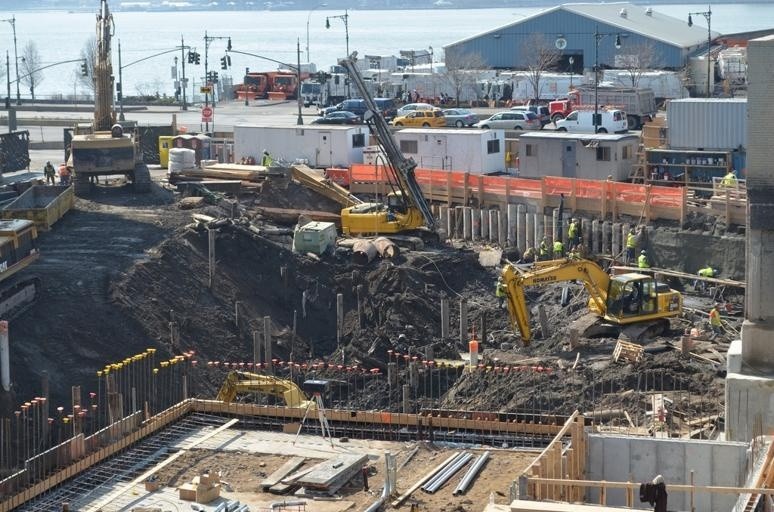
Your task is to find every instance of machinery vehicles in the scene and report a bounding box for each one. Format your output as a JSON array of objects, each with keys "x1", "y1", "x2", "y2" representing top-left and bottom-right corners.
[
  {"x1": 338, "y1": 51, "x2": 448, "y2": 251},
  {"x1": 217, "y1": 369, "x2": 317, "y2": 411},
  {"x1": 0, "y1": 218, "x2": 41, "y2": 323},
  {"x1": 503, "y1": 257, "x2": 684, "y2": 347},
  {"x1": 64, "y1": 0, "x2": 151, "y2": 195}
]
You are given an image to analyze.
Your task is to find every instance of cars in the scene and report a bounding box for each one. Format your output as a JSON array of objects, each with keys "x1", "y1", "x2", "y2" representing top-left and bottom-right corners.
[{"x1": 312, "y1": 98, "x2": 480, "y2": 128}]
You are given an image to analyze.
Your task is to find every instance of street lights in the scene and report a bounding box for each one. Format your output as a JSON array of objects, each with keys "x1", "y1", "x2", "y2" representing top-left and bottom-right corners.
[
  {"x1": 206, "y1": 37, "x2": 231, "y2": 131},
  {"x1": 569, "y1": 57, "x2": 574, "y2": 91},
  {"x1": 688, "y1": 12, "x2": 710, "y2": 98},
  {"x1": 16, "y1": 56, "x2": 25, "y2": 105},
  {"x1": 595, "y1": 33, "x2": 621, "y2": 134},
  {"x1": 307, "y1": 4, "x2": 327, "y2": 63},
  {"x1": 326, "y1": 15, "x2": 348, "y2": 56}
]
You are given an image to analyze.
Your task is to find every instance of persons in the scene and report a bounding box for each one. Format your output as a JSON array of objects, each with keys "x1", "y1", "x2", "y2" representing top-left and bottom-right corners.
[
  {"x1": 622, "y1": 283, "x2": 640, "y2": 313},
  {"x1": 538, "y1": 235, "x2": 550, "y2": 260},
  {"x1": 708, "y1": 302, "x2": 723, "y2": 344},
  {"x1": 383, "y1": 86, "x2": 450, "y2": 107},
  {"x1": 90, "y1": 176, "x2": 100, "y2": 185},
  {"x1": 636, "y1": 248, "x2": 650, "y2": 268},
  {"x1": 625, "y1": 224, "x2": 645, "y2": 263},
  {"x1": 44, "y1": 160, "x2": 57, "y2": 186},
  {"x1": 567, "y1": 217, "x2": 580, "y2": 253},
  {"x1": 716, "y1": 167, "x2": 742, "y2": 198},
  {"x1": 550, "y1": 238, "x2": 563, "y2": 259},
  {"x1": 58, "y1": 163, "x2": 71, "y2": 186},
  {"x1": 523, "y1": 247, "x2": 539, "y2": 265},
  {"x1": 495, "y1": 275, "x2": 507, "y2": 310},
  {"x1": 692, "y1": 265, "x2": 722, "y2": 293},
  {"x1": 260, "y1": 148, "x2": 270, "y2": 167}
]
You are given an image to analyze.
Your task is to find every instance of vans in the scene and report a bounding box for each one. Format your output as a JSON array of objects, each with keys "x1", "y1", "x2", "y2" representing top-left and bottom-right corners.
[
  {"x1": 556, "y1": 110, "x2": 628, "y2": 134},
  {"x1": 477, "y1": 105, "x2": 551, "y2": 130}
]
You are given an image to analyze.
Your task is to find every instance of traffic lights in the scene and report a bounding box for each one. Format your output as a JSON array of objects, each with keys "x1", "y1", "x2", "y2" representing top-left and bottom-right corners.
[
  {"x1": 221, "y1": 56, "x2": 227, "y2": 69},
  {"x1": 207, "y1": 71, "x2": 218, "y2": 83},
  {"x1": 188, "y1": 52, "x2": 200, "y2": 64},
  {"x1": 81, "y1": 63, "x2": 88, "y2": 77}
]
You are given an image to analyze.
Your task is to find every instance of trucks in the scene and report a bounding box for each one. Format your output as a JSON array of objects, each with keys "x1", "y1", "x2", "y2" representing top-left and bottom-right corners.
[
  {"x1": 244, "y1": 69, "x2": 352, "y2": 108},
  {"x1": 549, "y1": 87, "x2": 656, "y2": 130}
]
